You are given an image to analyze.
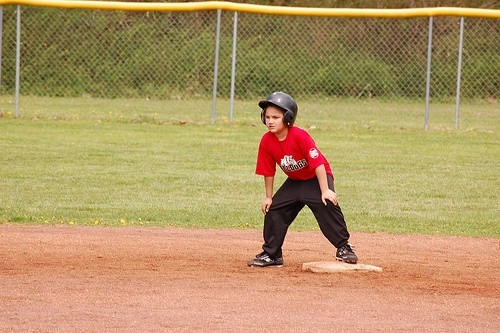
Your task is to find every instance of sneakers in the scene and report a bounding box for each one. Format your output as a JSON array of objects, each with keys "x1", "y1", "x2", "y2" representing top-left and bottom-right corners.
[
  {"x1": 336, "y1": 243, "x2": 359, "y2": 264},
  {"x1": 248, "y1": 251, "x2": 283, "y2": 267}
]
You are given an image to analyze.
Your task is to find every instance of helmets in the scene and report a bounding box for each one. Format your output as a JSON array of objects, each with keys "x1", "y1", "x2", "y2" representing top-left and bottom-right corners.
[{"x1": 258, "y1": 92, "x2": 298, "y2": 129}]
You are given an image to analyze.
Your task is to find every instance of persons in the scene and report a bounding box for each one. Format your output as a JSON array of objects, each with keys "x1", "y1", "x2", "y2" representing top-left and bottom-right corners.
[{"x1": 247, "y1": 91, "x2": 358, "y2": 266}]
